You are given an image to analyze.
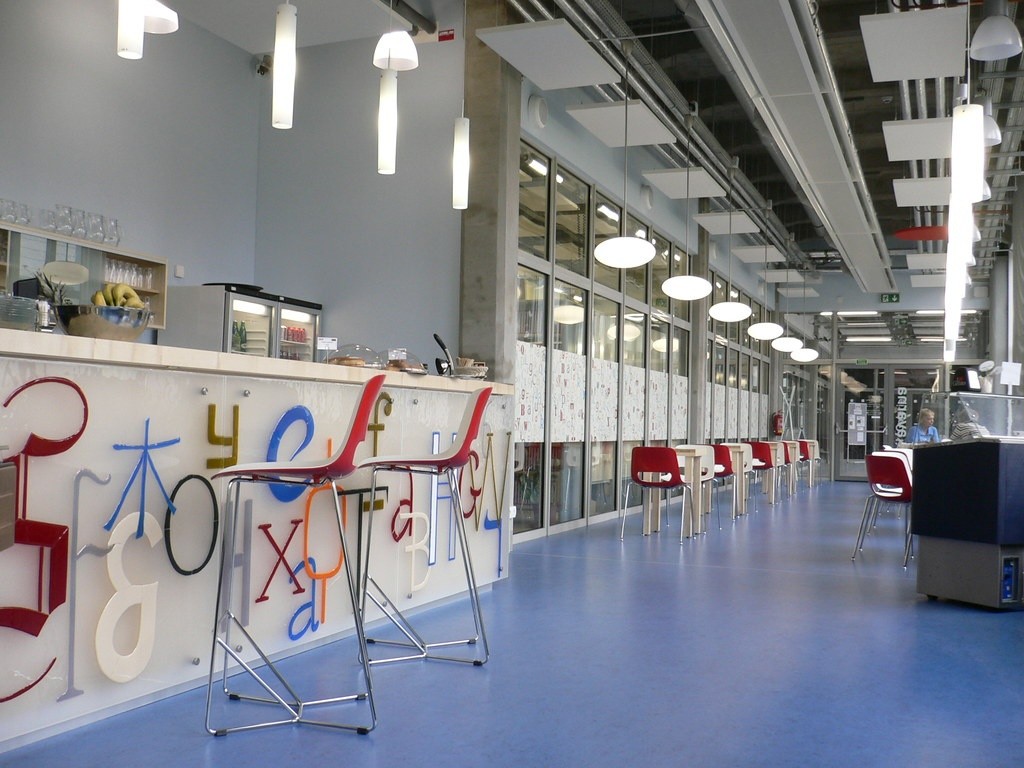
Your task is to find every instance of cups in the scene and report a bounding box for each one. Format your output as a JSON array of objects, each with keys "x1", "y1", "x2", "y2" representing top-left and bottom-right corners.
[{"x1": 0, "y1": 199, "x2": 120, "y2": 246}]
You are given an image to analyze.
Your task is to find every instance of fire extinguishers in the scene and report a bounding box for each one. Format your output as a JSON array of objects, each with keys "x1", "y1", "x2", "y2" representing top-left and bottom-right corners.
[{"x1": 772, "y1": 408, "x2": 784, "y2": 436}]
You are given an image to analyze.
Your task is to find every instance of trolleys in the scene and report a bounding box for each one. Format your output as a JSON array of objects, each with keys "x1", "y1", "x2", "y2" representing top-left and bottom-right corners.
[{"x1": 514, "y1": 442, "x2": 566, "y2": 530}]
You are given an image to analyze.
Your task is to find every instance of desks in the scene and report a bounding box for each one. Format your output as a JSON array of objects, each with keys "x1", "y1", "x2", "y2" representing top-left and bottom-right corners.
[
  {"x1": 805, "y1": 442, "x2": 816, "y2": 487},
  {"x1": 641, "y1": 448, "x2": 700, "y2": 539},
  {"x1": 777, "y1": 442, "x2": 797, "y2": 495},
  {"x1": 705, "y1": 444, "x2": 745, "y2": 514},
  {"x1": 746, "y1": 441, "x2": 778, "y2": 504}
]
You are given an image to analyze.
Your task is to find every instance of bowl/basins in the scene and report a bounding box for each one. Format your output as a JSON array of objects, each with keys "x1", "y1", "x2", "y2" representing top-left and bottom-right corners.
[
  {"x1": 52, "y1": 305, "x2": 154, "y2": 342},
  {"x1": 454, "y1": 367, "x2": 489, "y2": 376}
]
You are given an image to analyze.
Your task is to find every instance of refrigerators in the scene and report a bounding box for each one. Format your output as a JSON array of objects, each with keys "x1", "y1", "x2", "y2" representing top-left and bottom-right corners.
[{"x1": 157, "y1": 286, "x2": 323, "y2": 363}]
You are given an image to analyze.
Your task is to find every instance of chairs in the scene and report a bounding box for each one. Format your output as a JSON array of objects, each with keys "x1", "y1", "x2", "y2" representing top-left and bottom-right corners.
[
  {"x1": 852, "y1": 447, "x2": 914, "y2": 567},
  {"x1": 621, "y1": 440, "x2": 820, "y2": 542}
]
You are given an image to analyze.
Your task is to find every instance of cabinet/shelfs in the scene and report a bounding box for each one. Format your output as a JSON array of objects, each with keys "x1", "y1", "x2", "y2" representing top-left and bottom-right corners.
[{"x1": 0, "y1": 219, "x2": 168, "y2": 328}]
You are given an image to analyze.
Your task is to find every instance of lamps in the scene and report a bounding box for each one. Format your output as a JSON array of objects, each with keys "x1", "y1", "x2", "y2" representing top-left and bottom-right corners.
[
  {"x1": 117, "y1": 0, "x2": 146, "y2": 60},
  {"x1": 46, "y1": 243, "x2": 88, "y2": 286},
  {"x1": 942, "y1": 0, "x2": 1024, "y2": 362},
  {"x1": 594, "y1": 39, "x2": 655, "y2": 268},
  {"x1": 372, "y1": 33, "x2": 419, "y2": 70},
  {"x1": 453, "y1": 0, "x2": 469, "y2": 209},
  {"x1": 748, "y1": 208, "x2": 821, "y2": 362},
  {"x1": 550, "y1": 305, "x2": 680, "y2": 353},
  {"x1": 270, "y1": 1, "x2": 297, "y2": 128},
  {"x1": 663, "y1": 116, "x2": 712, "y2": 301},
  {"x1": 708, "y1": 167, "x2": 751, "y2": 323},
  {"x1": 143, "y1": 0, "x2": 179, "y2": 33},
  {"x1": 375, "y1": 0, "x2": 398, "y2": 175}
]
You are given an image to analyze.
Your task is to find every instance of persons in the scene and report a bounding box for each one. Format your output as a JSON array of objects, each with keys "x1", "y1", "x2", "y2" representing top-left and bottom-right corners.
[
  {"x1": 906, "y1": 408, "x2": 940, "y2": 443},
  {"x1": 949, "y1": 409, "x2": 990, "y2": 441}
]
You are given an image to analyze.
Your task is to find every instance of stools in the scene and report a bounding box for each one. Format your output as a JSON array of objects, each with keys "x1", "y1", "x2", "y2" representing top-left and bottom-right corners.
[
  {"x1": 354, "y1": 386, "x2": 494, "y2": 665},
  {"x1": 203, "y1": 374, "x2": 387, "y2": 737}
]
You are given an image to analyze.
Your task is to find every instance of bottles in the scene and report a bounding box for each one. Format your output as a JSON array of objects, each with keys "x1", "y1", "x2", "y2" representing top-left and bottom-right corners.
[
  {"x1": 232, "y1": 320, "x2": 247, "y2": 352},
  {"x1": 280, "y1": 346, "x2": 300, "y2": 360},
  {"x1": 105, "y1": 257, "x2": 153, "y2": 288}
]
